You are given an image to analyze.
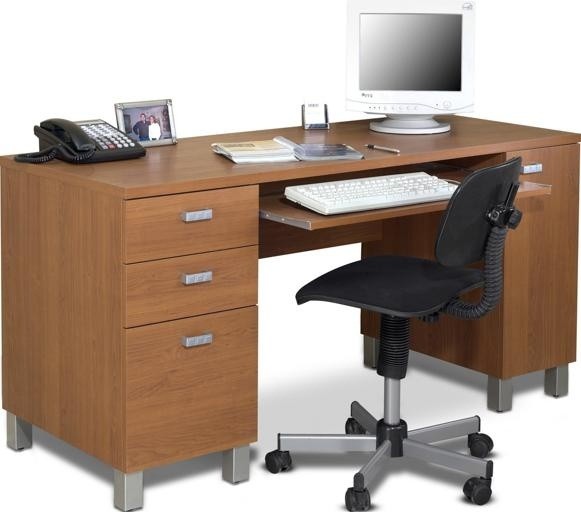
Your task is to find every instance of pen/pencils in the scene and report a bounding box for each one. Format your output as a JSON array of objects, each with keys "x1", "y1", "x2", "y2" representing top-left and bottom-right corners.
[{"x1": 365, "y1": 144, "x2": 401, "y2": 153}]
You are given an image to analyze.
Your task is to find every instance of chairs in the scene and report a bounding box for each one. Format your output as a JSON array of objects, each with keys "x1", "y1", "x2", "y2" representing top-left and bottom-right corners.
[{"x1": 266, "y1": 155, "x2": 526, "y2": 511}]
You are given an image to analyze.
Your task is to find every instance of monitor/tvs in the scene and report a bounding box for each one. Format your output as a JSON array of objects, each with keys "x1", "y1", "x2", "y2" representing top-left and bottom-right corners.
[{"x1": 346, "y1": 1, "x2": 478, "y2": 134}]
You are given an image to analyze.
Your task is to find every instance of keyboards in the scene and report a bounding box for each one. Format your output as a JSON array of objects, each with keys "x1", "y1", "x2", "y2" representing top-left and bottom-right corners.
[{"x1": 284, "y1": 170, "x2": 458, "y2": 217}]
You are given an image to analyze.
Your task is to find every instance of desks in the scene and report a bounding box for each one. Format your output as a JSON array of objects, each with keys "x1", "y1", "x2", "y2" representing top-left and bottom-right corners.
[{"x1": 1, "y1": 116, "x2": 581, "y2": 512}]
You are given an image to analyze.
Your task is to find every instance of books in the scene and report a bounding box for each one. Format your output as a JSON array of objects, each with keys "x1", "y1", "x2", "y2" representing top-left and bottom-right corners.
[
  {"x1": 300, "y1": 143, "x2": 365, "y2": 160},
  {"x1": 212, "y1": 136, "x2": 307, "y2": 164}
]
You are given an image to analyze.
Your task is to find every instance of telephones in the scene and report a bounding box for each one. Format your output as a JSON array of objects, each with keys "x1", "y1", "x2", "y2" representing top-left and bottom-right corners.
[{"x1": 31, "y1": 118, "x2": 146, "y2": 164}]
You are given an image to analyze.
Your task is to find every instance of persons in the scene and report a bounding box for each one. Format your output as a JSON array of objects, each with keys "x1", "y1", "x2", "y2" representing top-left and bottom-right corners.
[
  {"x1": 148, "y1": 115, "x2": 162, "y2": 141},
  {"x1": 133, "y1": 114, "x2": 158, "y2": 141}
]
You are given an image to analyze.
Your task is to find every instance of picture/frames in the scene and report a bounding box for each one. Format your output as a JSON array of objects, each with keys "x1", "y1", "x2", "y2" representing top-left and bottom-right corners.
[{"x1": 113, "y1": 98, "x2": 179, "y2": 148}]
